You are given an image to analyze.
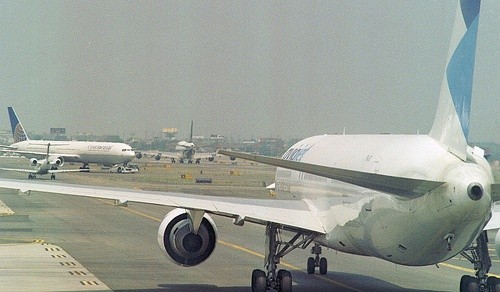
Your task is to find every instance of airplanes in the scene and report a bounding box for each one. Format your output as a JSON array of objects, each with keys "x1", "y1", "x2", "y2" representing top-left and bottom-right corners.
[
  {"x1": 0, "y1": 107, "x2": 136, "y2": 180},
  {"x1": 0, "y1": 0, "x2": 499, "y2": 292},
  {"x1": 134, "y1": 121, "x2": 236, "y2": 164}
]
